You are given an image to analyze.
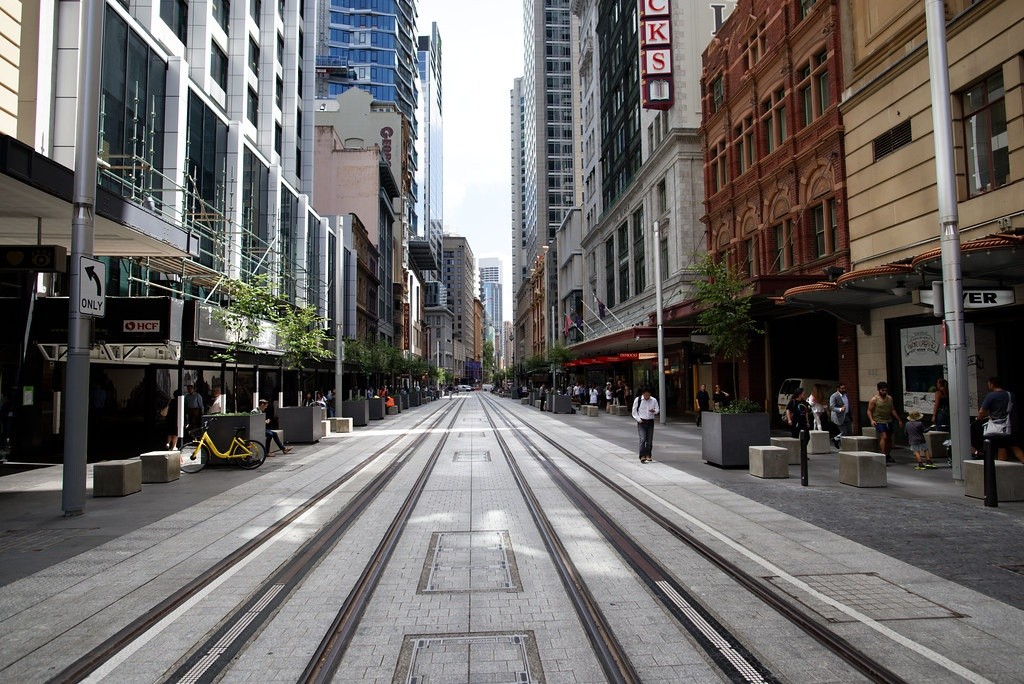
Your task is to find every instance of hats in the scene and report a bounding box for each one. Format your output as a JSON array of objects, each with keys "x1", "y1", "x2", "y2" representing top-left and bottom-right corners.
[{"x1": 907, "y1": 410, "x2": 923, "y2": 422}]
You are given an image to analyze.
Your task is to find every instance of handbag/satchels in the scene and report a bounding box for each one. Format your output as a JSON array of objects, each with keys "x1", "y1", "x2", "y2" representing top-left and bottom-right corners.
[
  {"x1": 160, "y1": 399, "x2": 170, "y2": 416},
  {"x1": 984, "y1": 417, "x2": 1011, "y2": 435},
  {"x1": 781, "y1": 399, "x2": 795, "y2": 422}
]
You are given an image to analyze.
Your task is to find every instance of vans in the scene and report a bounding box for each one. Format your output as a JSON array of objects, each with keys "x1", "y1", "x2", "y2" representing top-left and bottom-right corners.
[
  {"x1": 777, "y1": 377, "x2": 840, "y2": 429},
  {"x1": 481, "y1": 383, "x2": 494, "y2": 392}
]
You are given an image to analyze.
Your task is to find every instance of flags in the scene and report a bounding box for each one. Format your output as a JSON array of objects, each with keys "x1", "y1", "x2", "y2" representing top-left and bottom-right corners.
[
  {"x1": 573, "y1": 311, "x2": 582, "y2": 328},
  {"x1": 564, "y1": 315, "x2": 572, "y2": 335},
  {"x1": 598, "y1": 302, "x2": 605, "y2": 319}
]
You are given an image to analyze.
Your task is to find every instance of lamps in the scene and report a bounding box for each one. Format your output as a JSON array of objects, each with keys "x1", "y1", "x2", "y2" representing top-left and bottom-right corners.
[
  {"x1": 144, "y1": 197, "x2": 155, "y2": 211},
  {"x1": 320, "y1": 105, "x2": 325, "y2": 111},
  {"x1": 891, "y1": 280, "x2": 908, "y2": 296}
]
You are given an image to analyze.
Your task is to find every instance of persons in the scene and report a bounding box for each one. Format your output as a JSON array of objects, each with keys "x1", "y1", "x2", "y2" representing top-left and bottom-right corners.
[
  {"x1": 162, "y1": 389, "x2": 189, "y2": 451},
  {"x1": 905, "y1": 411, "x2": 936, "y2": 469},
  {"x1": 539, "y1": 383, "x2": 545, "y2": 410},
  {"x1": 185, "y1": 385, "x2": 204, "y2": 445},
  {"x1": 712, "y1": 385, "x2": 729, "y2": 411},
  {"x1": 366, "y1": 385, "x2": 394, "y2": 407},
  {"x1": 695, "y1": 384, "x2": 710, "y2": 427},
  {"x1": 632, "y1": 388, "x2": 660, "y2": 462},
  {"x1": 447, "y1": 384, "x2": 453, "y2": 400},
  {"x1": 808, "y1": 384, "x2": 829, "y2": 431},
  {"x1": 867, "y1": 382, "x2": 902, "y2": 463},
  {"x1": 250, "y1": 399, "x2": 292, "y2": 457},
  {"x1": 932, "y1": 378, "x2": 950, "y2": 426},
  {"x1": 301, "y1": 389, "x2": 336, "y2": 417},
  {"x1": 558, "y1": 380, "x2": 630, "y2": 414},
  {"x1": 830, "y1": 384, "x2": 853, "y2": 449},
  {"x1": 786, "y1": 388, "x2": 811, "y2": 459},
  {"x1": 208, "y1": 385, "x2": 222, "y2": 413},
  {"x1": 969, "y1": 377, "x2": 1024, "y2": 465}
]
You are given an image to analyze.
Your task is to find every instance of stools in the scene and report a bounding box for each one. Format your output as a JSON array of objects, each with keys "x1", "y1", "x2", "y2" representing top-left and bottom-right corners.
[
  {"x1": 963, "y1": 460, "x2": 1024, "y2": 502},
  {"x1": 610, "y1": 405, "x2": 628, "y2": 415},
  {"x1": 749, "y1": 427, "x2": 950, "y2": 487},
  {"x1": 521, "y1": 397, "x2": 546, "y2": 410},
  {"x1": 581, "y1": 405, "x2": 598, "y2": 416},
  {"x1": 94, "y1": 451, "x2": 180, "y2": 496},
  {"x1": 265, "y1": 430, "x2": 284, "y2": 453},
  {"x1": 321, "y1": 407, "x2": 353, "y2": 436}
]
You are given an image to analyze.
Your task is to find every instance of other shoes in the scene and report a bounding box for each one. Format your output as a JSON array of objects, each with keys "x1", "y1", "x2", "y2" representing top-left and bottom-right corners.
[
  {"x1": 265, "y1": 452, "x2": 275, "y2": 457},
  {"x1": 166, "y1": 443, "x2": 170, "y2": 449},
  {"x1": 171, "y1": 447, "x2": 178, "y2": 451},
  {"x1": 886, "y1": 457, "x2": 895, "y2": 463},
  {"x1": 925, "y1": 462, "x2": 937, "y2": 468},
  {"x1": 282, "y1": 447, "x2": 293, "y2": 454},
  {"x1": 641, "y1": 456, "x2": 647, "y2": 463},
  {"x1": 914, "y1": 465, "x2": 926, "y2": 470},
  {"x1": 831, "y1": 438, "x2": 840, "y2": 449},
  {"x1": 645, "y1": 455, "x2": 652, "y2": 461}
]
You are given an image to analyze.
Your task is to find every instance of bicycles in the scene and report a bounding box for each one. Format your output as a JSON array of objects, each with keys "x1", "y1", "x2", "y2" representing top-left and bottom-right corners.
[{"x1": 179, "y1": 416, "x2": 267, "y2": 474}]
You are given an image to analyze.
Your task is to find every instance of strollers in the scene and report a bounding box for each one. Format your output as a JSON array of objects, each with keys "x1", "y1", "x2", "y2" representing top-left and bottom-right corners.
[{"x1": 944, "y1": 415, "x2": 991, "y2": 467}]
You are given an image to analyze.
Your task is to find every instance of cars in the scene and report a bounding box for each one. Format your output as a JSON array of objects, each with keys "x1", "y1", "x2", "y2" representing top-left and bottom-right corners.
[{"x1": 457, "y1": 384, "x2": 473, "y2": 391}]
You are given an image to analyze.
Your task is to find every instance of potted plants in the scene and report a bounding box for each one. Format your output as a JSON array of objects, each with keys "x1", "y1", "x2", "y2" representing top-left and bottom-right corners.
[
  {"x1": 342, "y1": 338, "x2": 446, "y2": 427},
  {"x1": 201, "y1": 273, "x2": 337, "y2": 469},
  {"x1": 546, "y1": 342, "x2": 577, "y2": 412},
  {"x1": 686, "y1": 248, "x2": 770, "y2": 470}
]
